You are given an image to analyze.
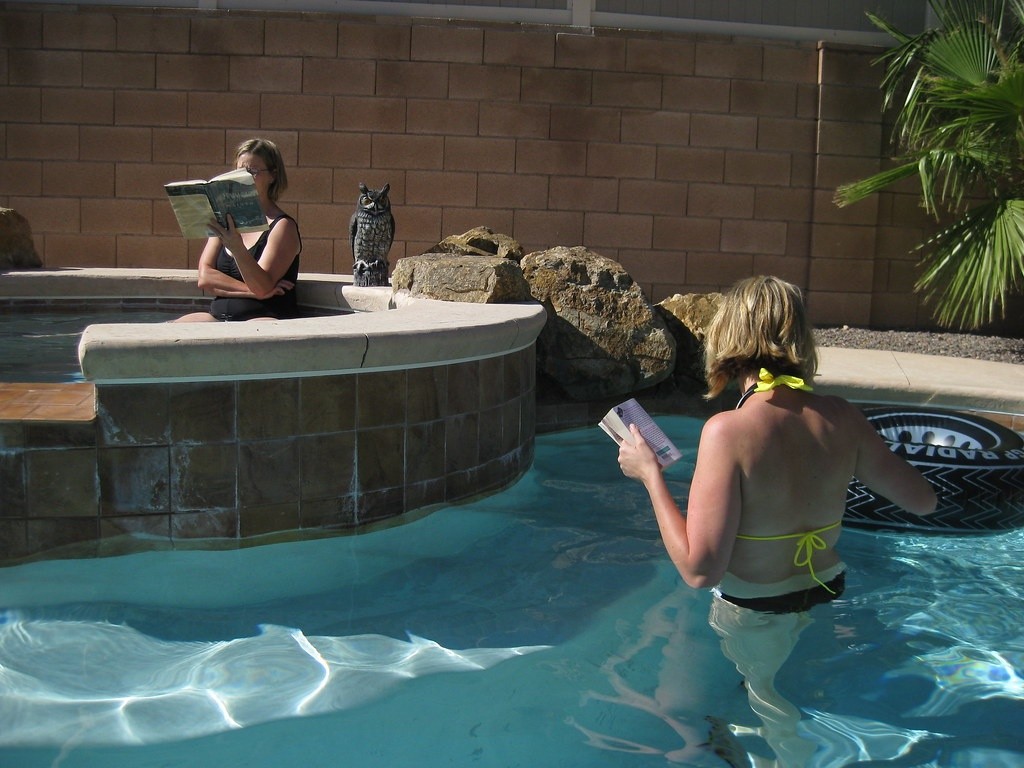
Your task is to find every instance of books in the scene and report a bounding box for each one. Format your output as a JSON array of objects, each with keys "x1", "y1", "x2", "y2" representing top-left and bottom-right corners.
[
  {"x1": 598, "y1": 398, "x2": 683, "y2": 472},
  {"x1": 163, "y1": 168, "x2": 270, "y2": 240}
]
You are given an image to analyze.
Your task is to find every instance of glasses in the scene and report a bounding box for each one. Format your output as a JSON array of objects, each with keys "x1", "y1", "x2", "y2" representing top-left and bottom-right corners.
[{"x1": 248, "y1": 166, "x2": 271, "y2": 177}]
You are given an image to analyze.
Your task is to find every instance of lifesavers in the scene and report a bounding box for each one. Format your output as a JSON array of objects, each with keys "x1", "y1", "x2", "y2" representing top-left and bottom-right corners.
[{"x1": 841, "y1": 405, "x2": 1024, "y2": 538}]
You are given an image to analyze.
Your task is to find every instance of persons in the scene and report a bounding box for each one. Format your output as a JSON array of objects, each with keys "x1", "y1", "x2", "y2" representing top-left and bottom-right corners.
[
  {"x1": 618, "y1": 276, "x2": 938, "y2": 615},
  {"x1": 172, "y1": 138, "x2": 301, "y2": 322}
]
